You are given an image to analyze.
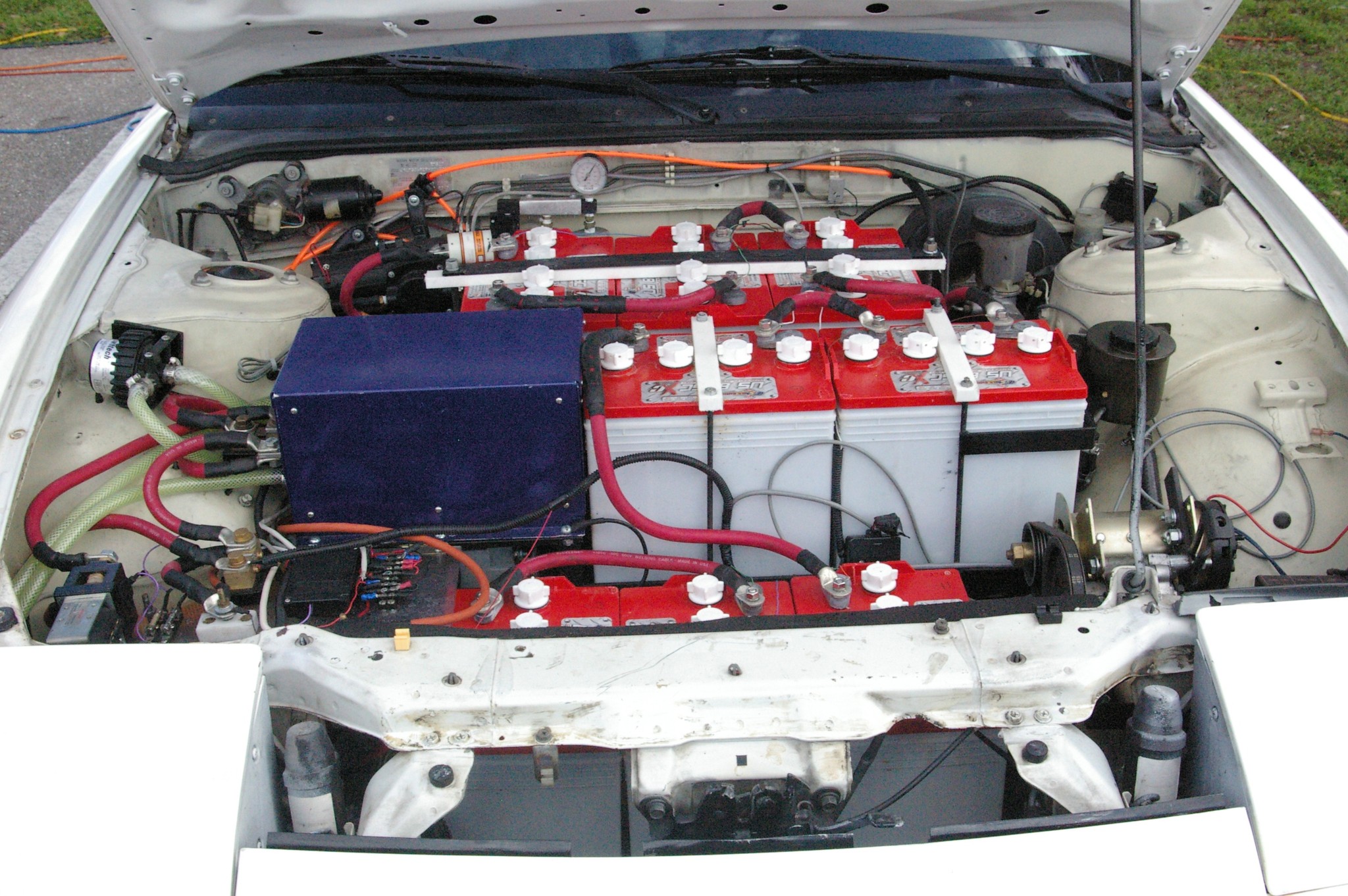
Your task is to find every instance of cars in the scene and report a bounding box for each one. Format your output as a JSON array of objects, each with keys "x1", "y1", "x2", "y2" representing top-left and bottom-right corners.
[{"x1": 0, "y1": 1, "x2": 1348, "y2": 895}]
